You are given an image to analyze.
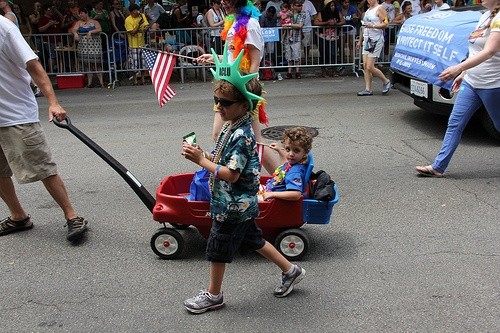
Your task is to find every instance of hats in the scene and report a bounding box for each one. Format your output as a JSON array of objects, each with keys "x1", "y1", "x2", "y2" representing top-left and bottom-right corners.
[{"x1": 324, "y1": 0, "x2": 334, "y2": 8}]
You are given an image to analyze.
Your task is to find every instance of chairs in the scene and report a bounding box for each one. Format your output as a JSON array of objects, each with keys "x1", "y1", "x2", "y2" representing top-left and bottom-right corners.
[{"x1": 179, "y1": 45, "x2": 206, "y2": 83}]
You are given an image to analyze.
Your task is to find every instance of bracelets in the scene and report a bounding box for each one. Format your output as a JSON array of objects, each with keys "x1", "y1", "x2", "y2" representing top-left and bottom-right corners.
[
  {"x1": 373, "y1": 25, "x2": 375, "y2": 27},
  {"x1": 289, "y1": 23, "x2": 291, "y2": 26}
]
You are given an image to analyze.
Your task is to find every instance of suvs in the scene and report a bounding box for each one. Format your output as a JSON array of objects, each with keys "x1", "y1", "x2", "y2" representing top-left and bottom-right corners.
[{"x1": 389, "y1": 4, "x2": 500, "y2": 127}]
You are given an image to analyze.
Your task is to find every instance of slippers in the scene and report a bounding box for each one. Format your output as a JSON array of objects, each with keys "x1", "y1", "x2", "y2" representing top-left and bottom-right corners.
[{"x1": 414, "y1": 164, "x2": 437, "y2": 177}]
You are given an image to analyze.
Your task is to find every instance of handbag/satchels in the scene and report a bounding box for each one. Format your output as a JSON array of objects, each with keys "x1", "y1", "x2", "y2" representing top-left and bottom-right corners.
[{"x1": 364, "y1": 38, "x2": 376, "y2": 53}]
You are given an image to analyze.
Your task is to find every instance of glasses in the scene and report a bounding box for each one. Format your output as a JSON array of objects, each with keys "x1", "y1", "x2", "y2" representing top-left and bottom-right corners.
[
  {"x1": 13, "y1": 7, "x2": 19, "y2": 9},
  {"x1": 213, "y1": 95, "x2": 246, "y2": 107}
]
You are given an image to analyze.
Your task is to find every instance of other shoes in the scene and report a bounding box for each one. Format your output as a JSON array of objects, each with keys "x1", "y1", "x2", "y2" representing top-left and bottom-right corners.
[
  {"x1": 295, "y1": 73, "x2": 300, "y2": 78},
  {"x1": 84, "y1": 85, "x2": 92, "y2": 88},
  {"x1": 286, "y1": 73, "x2": 291, "y2": 79},
  {"x1": 34, "y1": 86, "x2": 42, "y2": 96},
  {"x1": 101, "y1": 84, "x2": 105, "y2": 88}
]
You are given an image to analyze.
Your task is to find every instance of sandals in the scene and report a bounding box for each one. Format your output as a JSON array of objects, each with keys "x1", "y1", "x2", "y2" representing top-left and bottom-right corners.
[
  {"x1": 64, "y1": 217, "x2": 88, "y2": 240},
  {"x1": 0, "y1": 214, "x2": 33, "y2": 236}
]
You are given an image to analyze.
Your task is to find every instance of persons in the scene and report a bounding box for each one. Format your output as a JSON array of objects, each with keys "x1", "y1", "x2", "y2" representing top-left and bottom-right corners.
[
  {"x1": 0, "y1": 0, "x2": 481, "y2": 96},
  {"x1": 257, "y1": 127, "x2": 313, "y2": 201},
  {"x1": 181, "y1": 42, "x2": 307, "y2": 314},
  {"x1": 192, "y1": 1, "x2": 283, "y2": 174},
  {"x1": 415, "y1": 0, "x2": 500, "y2": 177},
  {"x1": 0, "y1": 15, "x2": 89, "y2": 241}
]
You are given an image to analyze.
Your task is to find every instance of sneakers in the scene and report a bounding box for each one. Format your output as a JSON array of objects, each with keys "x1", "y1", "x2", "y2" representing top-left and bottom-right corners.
[
  {"x1": 358, "y1": 90, "x2": 374, "y2": 96},
  {"x1": 183, "y1": 290, "x2": 224, "y2": 314},
  {"x1": 382, "y1": 78, "x2": 390, "y2": 95},
  {"x1": 274, "y1": 267, "x2": 306, "y2": 298}
]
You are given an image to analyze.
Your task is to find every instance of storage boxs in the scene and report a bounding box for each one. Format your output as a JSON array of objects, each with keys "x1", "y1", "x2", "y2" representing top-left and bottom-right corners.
[{"x1": 56, "y1": 74, "x2": 84, "y2": 89}]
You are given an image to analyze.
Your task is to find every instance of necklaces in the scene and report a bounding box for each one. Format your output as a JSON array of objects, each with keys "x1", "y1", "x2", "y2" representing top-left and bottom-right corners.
[{"x1": 207, "y1": 111, "x2": 251, "y2": 188}]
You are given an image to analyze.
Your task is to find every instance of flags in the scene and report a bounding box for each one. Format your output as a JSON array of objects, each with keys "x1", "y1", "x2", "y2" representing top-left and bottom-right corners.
[{"x1": 139, "y1": 46, "x2": 178, "y2": 107}]
